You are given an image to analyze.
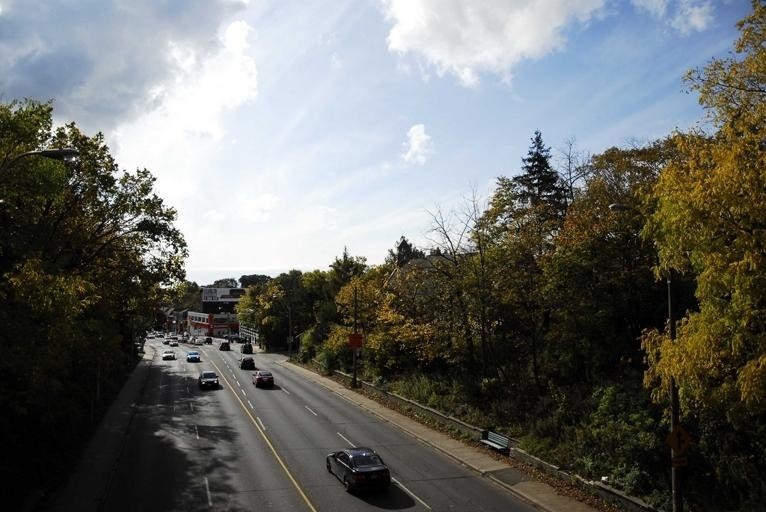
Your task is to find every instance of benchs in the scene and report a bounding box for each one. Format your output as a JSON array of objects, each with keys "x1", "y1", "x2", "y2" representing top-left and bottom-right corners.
[{"x1": 479, "y1": 430, "x2": 510, "y2": 458}]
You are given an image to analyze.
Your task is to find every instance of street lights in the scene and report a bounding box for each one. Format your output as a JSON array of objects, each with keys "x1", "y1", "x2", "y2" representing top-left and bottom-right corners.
[
  {"x1": 328, "y1": 264, "x2": 356, "y2": 387},
  {"x1": 606, "y1": 202, "x2": 684, "y2": 511}
]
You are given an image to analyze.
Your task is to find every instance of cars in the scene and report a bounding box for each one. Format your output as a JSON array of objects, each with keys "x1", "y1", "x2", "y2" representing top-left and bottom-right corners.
[
  {"x1": 185, "y1": 351, "x2": 199, "y2": 362},
  {"x1": 161, "y1": 349, "x2": 175, "y2": 360},
  {"x1": 197, "y1": 371, "x2": 218, "y2": 389},
  {"x1": 326, "y1": 447, "x2": 390, "y2": 493},
  {"x1": 251, "y1": 371, "x2": 273, "y2": 388},
  {"x1": 237, "y1": 357, "x2": 254, "y2": 370},
  {"x1": 218, "y1": 342, "x2": 229, "y2": 351},
  {"x1": 240, "y1": 344, "x2": 252, "y2": 353},
  {"x1": 145, "y1": 330, "x2": 211, "y2": 346}
]
